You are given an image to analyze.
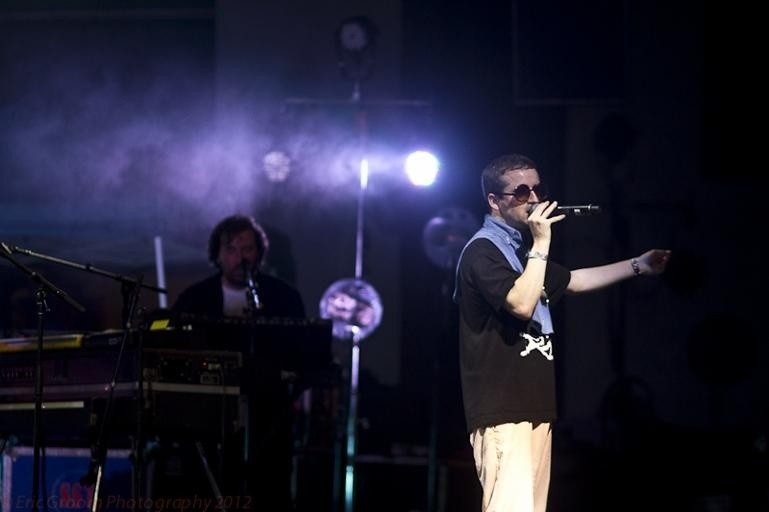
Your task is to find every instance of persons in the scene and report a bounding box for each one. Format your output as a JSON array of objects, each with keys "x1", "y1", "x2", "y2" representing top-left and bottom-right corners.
[
  {"x1": 451, "y1": 147, "x2": 674, "y2": 512},
  {"x1": 151, "y1": 209, "x2": 310, "y2": 392}
]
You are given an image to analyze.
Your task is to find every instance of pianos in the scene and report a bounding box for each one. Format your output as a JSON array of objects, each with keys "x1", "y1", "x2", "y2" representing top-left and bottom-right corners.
[{"x1": 0, "y1": 325, "x2": 244, "y2": 403}]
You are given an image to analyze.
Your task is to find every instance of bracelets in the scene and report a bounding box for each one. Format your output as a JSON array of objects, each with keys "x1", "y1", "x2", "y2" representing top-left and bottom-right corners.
[{"x1": 629, "y1": 255, "x2": 641, "y2": 280}]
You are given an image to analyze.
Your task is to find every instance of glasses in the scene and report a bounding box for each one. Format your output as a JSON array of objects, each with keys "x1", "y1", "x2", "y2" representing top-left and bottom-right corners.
[{"x1": 499, "y1": 183, "x2": 548, "y2": 203}]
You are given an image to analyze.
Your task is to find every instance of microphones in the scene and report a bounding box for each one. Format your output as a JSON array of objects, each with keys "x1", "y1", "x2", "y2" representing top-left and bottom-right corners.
[
  {"x1": 241, "y1": 258, "x2": 260, "y2": 310},
  {"x1": 528, "y1": 203, "x2": 599, "y2": 215}
]
